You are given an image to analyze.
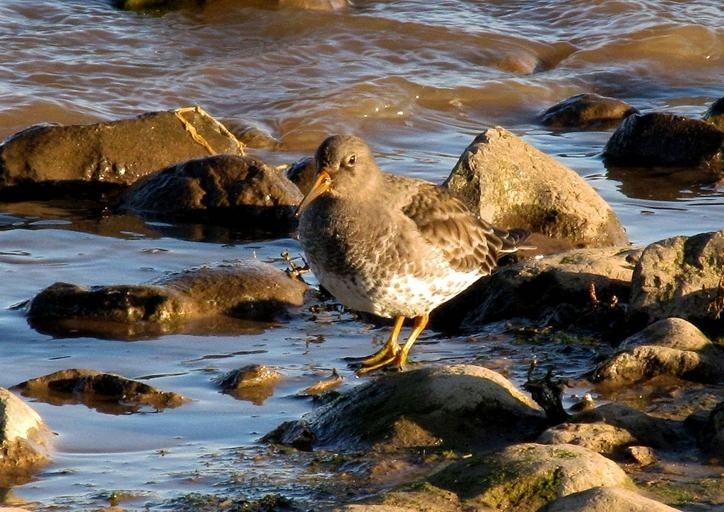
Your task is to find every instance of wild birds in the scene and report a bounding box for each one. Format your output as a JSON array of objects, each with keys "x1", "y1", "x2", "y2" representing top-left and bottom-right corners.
[{"x1": 295, "y1": 135, "x2": 539, "y2": 376}]
